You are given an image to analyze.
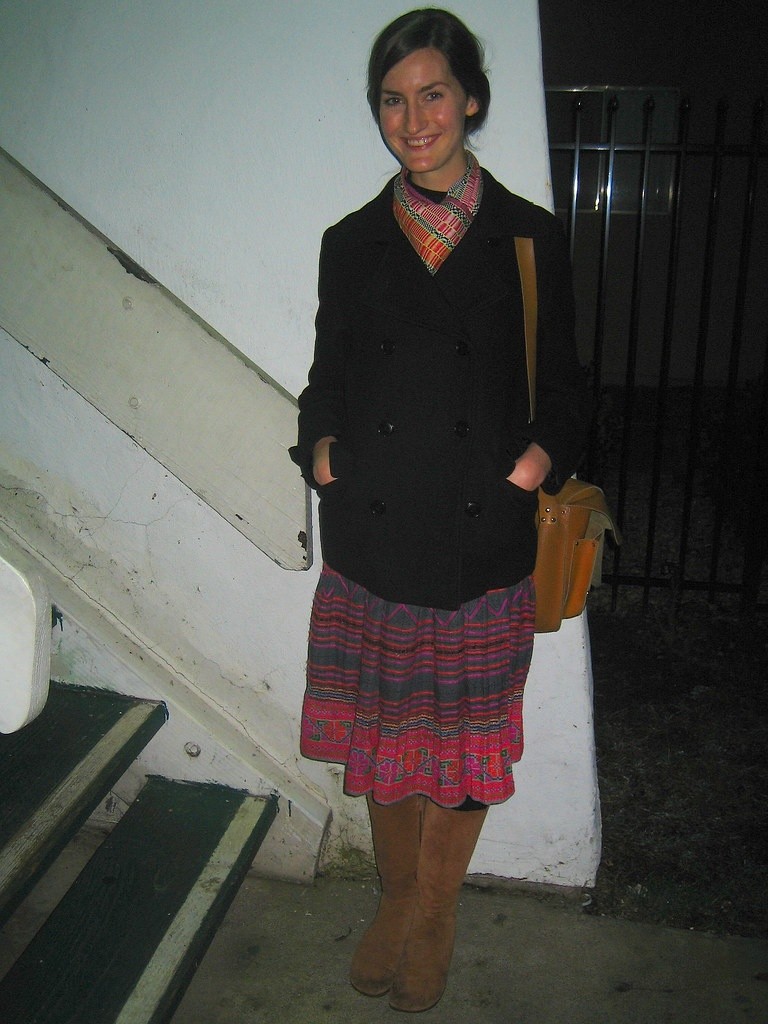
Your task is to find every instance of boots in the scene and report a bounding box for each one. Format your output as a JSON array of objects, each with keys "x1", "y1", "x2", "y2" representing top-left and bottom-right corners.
[
  {"x1": 388, "y1": 798, "x2": 502, "y2": 1012},
  {"x1": 350, "y1": 788, "x2": 426, "y2": 995}
]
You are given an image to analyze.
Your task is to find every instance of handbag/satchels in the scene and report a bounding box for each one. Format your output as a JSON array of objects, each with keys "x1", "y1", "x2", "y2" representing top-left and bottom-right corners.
[{"x1": 529, "y1": 475, "x2": 620, "y2": 632}]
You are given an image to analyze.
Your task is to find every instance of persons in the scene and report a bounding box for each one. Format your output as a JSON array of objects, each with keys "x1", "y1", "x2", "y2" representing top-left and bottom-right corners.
[{"x1": 299, "y1": 9, "x2": 584, "y2": 1012}]
